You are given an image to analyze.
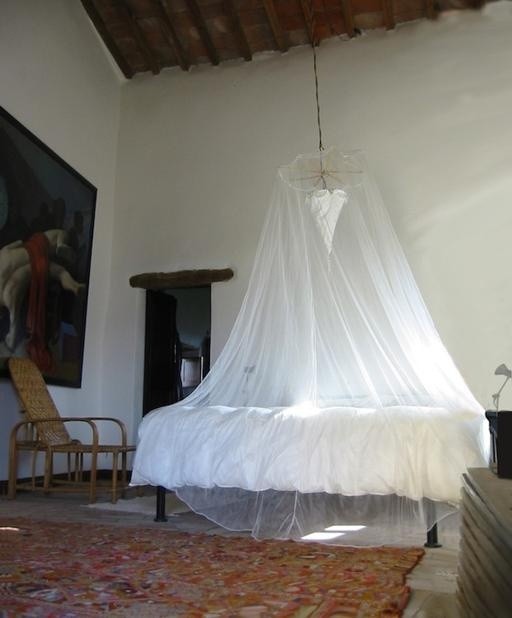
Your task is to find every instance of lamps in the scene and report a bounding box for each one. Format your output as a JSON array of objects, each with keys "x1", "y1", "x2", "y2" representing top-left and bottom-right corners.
[
  {"x1": 300, "y1": 0, "x2": 347, "y2": 257},
  {"x1": 490, "y1": 363, "x2": 511, "y2": 413}
]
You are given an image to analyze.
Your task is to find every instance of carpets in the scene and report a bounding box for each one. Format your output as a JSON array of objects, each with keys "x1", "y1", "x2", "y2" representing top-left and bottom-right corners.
[
  {"x1": 86, "y1": 487, "x2": 246, "y2": 520},
  {"x1": 1, "y1": 512, "x2": 433, "y2": 618}
]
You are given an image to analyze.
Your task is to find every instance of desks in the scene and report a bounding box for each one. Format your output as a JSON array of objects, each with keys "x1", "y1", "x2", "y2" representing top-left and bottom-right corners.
[{"x1": 452, "y1": 467, "x2": 511, "y2": 618}]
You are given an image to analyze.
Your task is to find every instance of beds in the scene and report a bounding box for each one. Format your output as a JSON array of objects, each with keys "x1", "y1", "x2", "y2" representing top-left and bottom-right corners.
[{"x1": 128, "y1": 396, "x2": 495, "y2": 552}]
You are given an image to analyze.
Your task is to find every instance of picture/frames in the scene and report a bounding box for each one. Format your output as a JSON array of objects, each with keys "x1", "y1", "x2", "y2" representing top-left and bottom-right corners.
[{"x1": 1, "y1": 103, "x2": 103, "y2": 392}]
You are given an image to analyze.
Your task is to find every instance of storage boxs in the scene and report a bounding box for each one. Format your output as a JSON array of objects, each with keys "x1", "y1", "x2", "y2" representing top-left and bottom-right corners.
[{"x1": 483, "y1": 405, "x2": 511, "y2": 478}]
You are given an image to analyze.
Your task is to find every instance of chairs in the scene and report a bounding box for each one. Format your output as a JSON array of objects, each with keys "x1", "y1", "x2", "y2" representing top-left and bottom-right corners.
[{"x1": 5, "y1": 358, "x2": 132, "y2": 504}]
